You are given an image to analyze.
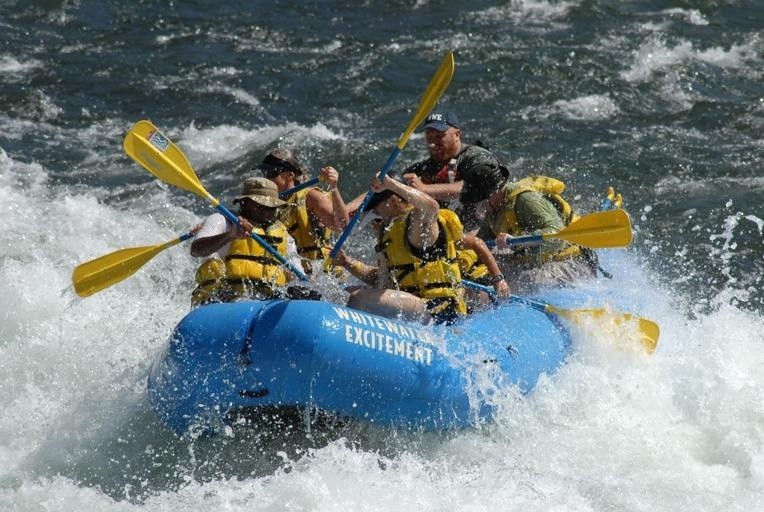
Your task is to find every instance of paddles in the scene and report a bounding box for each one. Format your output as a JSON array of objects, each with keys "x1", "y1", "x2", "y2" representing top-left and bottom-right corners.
[
  {"x1": 462, "y1": 281, "x2": 660, "y2": 356},
  {"x1": 72, "y1": 172, "x2": 324, "y2": 297},
  {"x1": 322, "y1": 52, "x2": 456, "y2": 274},
  {"x1": 122, "y1": 120, "x2": 313, "y2": 284},
  {"x1": 485, "y1": 209, "x2": 633, "y2": 248}
]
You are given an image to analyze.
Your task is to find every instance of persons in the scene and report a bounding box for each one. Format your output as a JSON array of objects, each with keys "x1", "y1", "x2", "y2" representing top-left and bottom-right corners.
[{"x1": 189, "y1": 108, "x2": 598, "y2": 327}]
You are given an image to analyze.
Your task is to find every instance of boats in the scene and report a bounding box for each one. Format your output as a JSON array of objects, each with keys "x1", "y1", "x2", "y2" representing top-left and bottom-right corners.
[{"x1": 142, "y1": 200, "x2": 699, "y2": 458}]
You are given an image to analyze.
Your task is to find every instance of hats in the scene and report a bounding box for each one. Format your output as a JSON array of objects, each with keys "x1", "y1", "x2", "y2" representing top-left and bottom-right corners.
[
  {"x1": 251, "y1": 146, "x2": 302, "y2": 176},
  {"x1": 233, "y1": 177, "x2": 288, "y2": 209},
  {"x1": 459, "y1": 163, "x2": 509, "y2": 202},
  {"x1": 363, "y1": 171, "x2": 408, "y2": 212},
  {"x1": 422, "y1": 108, "x2": 460, "y2": 133}
]
